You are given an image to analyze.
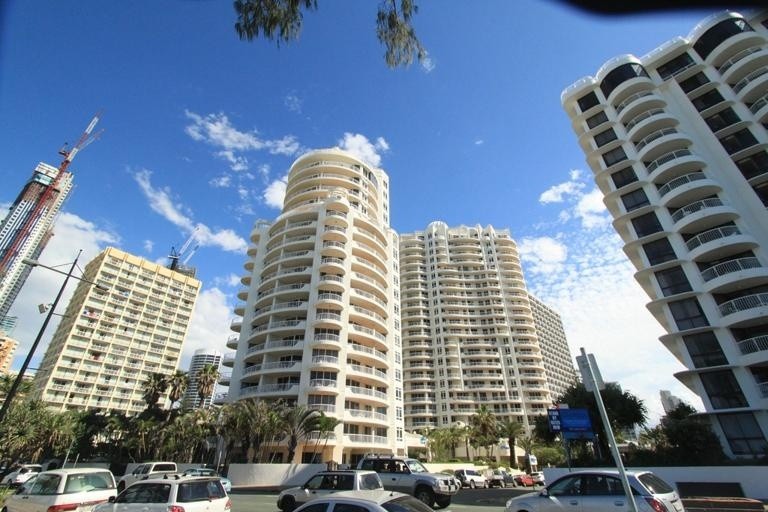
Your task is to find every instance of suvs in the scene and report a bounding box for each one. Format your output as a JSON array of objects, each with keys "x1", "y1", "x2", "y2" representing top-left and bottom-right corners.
[{"x1": 357, "y1": 452, "x2": 462, "y2": 508}]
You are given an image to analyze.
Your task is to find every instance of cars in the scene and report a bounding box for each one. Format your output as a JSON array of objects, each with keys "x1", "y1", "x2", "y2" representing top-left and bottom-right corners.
[
  {"x1": 505, "y1": 471, "x2": 685, "y2": 511},
  {"x1": 2, "y1": 468, "x2": 117, "y2": 512},
  {"x1": 455, "y1": 469, "x2": 546, "y2": 489},
  {"x1": 277, "y1": 470, "x2": 384, "y2": 511},
  {"x1": 293, "y1": 490, "x2": 436, "y2": 512},
  {"x1": 92, "y1": 462, "x2": 232, "y2": 512},
  {"x1": 1, "y1": 464, "x2": 42, "y2": 489}
]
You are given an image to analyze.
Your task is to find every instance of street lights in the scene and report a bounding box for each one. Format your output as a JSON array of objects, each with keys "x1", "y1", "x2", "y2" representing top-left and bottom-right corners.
[{"x1": 1, "y1": 250, "x2": 109, "y2": 421}]
